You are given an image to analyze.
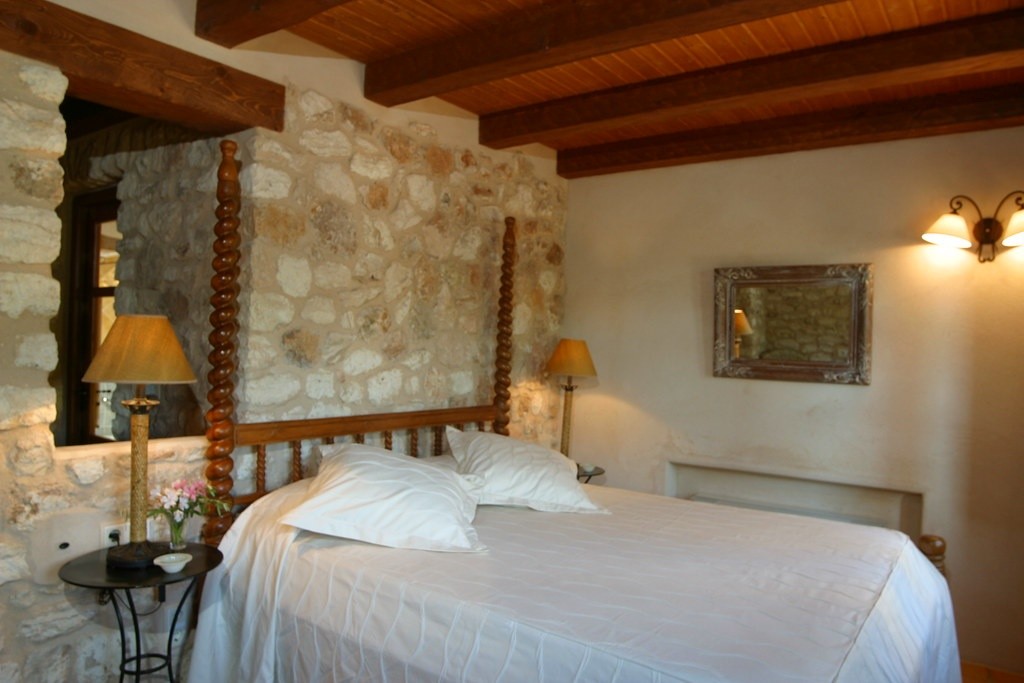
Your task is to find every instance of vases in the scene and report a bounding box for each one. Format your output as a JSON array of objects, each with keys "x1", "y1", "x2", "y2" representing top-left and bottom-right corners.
[{"x1": 167, "y1": 516, "x2": 188, "y2": 550}]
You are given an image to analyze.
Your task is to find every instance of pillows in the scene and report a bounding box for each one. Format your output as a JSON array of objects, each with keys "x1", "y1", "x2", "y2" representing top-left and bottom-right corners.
[
  {"x1": 276, "y1": 443, "x2": 489, "y2": 553},
  {"x1": 445, "y1": 425, "x2": 610, "y2": 515}
]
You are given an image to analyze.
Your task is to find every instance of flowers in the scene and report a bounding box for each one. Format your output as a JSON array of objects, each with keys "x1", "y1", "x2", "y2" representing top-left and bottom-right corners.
[{"x1": 141, "y1": 478, "x2": 230, "y2": 521}]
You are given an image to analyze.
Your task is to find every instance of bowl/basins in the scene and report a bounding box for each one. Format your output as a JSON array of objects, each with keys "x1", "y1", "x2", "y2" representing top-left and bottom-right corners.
[
  {"x1": 153, "y1": 553, "x2": 193, "y2": 574},
  {"x1": 580, "y1": 464, "x2": 596, "y2": 473}
]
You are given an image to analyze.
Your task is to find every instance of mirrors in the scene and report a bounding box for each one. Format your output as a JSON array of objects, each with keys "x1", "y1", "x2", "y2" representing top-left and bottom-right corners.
[{"x1": 712, "y1": 264, "x2": 873, "y2": 384}]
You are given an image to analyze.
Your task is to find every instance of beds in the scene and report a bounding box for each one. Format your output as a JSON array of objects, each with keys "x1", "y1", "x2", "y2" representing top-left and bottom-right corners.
[{"x1": 199, "y1": 141, "x2": 962, "y2": 683}]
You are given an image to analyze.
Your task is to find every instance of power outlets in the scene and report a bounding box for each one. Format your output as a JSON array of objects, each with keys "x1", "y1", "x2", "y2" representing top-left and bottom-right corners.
[{"x1": 103, "y1": 524, "x2": 129, "y2": 546}]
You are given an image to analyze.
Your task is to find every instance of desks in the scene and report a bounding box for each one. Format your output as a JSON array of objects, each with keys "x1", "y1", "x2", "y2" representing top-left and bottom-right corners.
[
  {"x1": 58, "y1": 542, "x2": 224, "y2": 683},
  {"x1": 577, "y1": 465, "x2": 605, "y2": 483}
]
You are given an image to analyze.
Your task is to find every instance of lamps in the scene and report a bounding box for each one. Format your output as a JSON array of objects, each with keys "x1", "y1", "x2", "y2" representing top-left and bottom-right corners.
[
  {"x1": 82, "y1": 315, "x2": 198, "y2": 575},
  {"x1": 732, "y1": 310, "x2": 752, "y2": 359},
  {"x1": 543, "y1": 339, "x2": 596, "y2": 475},
  {"x1": 922, "y1": 190, "x2": 1024, "y2": 263}
]
